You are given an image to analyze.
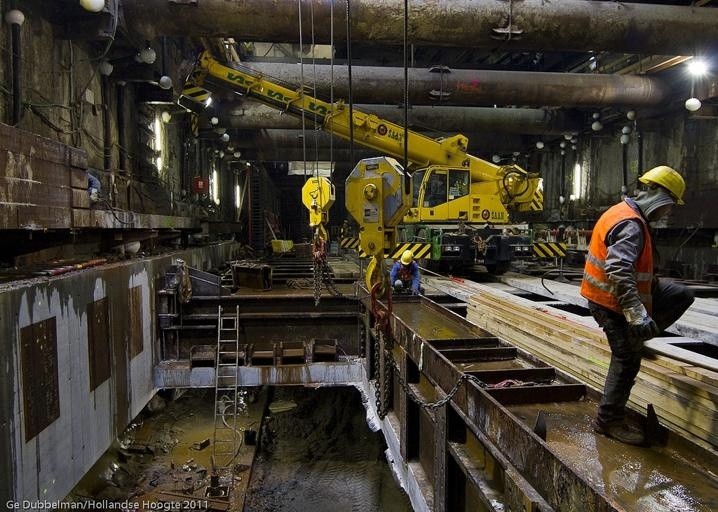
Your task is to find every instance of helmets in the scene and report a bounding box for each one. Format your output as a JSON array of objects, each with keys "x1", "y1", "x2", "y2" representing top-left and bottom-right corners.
[
  {"x1": 401, "y1": 250, "x2": 413, "y2": 265},
  {"x1": 638, "y1": 165, "x2": 686, "y2": 206}
]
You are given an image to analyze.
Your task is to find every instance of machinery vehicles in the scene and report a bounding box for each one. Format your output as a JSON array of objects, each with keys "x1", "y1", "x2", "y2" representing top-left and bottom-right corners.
[{"x1": 173, "y1": 46, "x2": 546, "y2": 277}]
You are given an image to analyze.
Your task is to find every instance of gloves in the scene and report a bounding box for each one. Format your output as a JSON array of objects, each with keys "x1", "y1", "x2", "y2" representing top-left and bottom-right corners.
[
  {"x1": 622, "y1": 303, "x2": 659, "y2": 340},
  {"x1": 394, "y1": 279, "x2": 403, "y2": 287},
  {"x1": 410, "y1": 287, "x2": 421, "y2": 295}
]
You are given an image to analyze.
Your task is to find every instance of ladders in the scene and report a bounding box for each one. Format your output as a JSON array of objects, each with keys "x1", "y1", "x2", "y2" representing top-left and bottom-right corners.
[{"x1": 213, "y1": 306, "x2": 238, "y2": 471}]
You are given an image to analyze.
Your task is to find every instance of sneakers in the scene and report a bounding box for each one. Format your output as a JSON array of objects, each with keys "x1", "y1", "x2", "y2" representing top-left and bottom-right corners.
[{"x1": 592, "y1": 417, "x2": 644, "y2": 444}]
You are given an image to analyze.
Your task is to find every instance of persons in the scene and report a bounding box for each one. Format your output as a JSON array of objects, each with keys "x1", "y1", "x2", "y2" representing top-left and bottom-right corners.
[
  {"x1": 579, "y1": 165, "x2": 698, "y2": 445},
  {"x1": 390, "y1": 248, "x2": 423, "y2": 298}
]
